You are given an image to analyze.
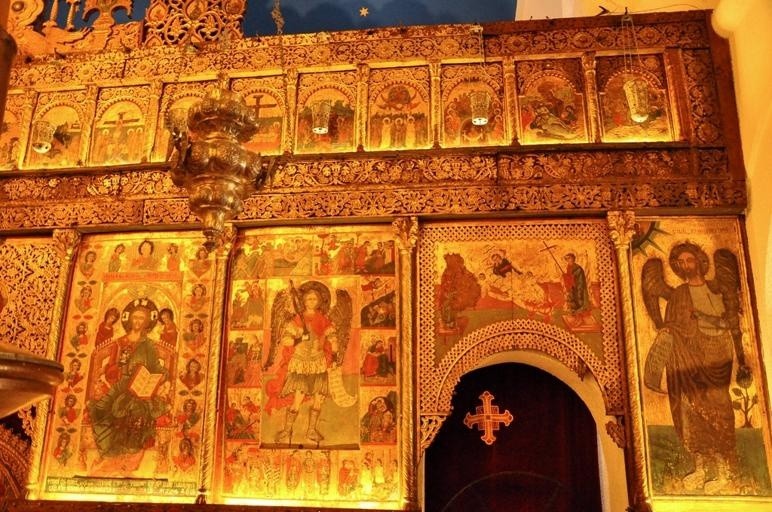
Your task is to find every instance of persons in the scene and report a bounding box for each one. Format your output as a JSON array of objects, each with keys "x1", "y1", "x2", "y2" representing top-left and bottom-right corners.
[
  {"x1": 561, "y1": 252, "x2": 587, "y2": 319},
  {"x1": 395, "y1": 118, "x2": 404, "y2": 147},
  {"x1": 405, "y1": 116, "x2": 416, "y2": 147},
  {"x1": 94, "y1": 125, "x2": 143, "y2": 165},
  {"x1": 443, "y1": 89, "x2": 503, "y2": 143},
  {"x1": 653, "y1": 238, "x2": 749, "y2": 493},
  {"x1": 562, "y1": 105, "x2": 578, "y2": 122},
  {"x1": 380, "y1": 118, "x2": 392, "y2": 148},
  {"x1": 487, "y1": 250, "x2": 521, "y2": 275},
  {"x1": 475, "y1": 272, "x2": 491, "y2": 300}
]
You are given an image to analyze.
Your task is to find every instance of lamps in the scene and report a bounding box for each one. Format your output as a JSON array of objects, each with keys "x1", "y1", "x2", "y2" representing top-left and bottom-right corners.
[{"x1": 165, "y1": 0, "x2": 294, "y2": 256}]
